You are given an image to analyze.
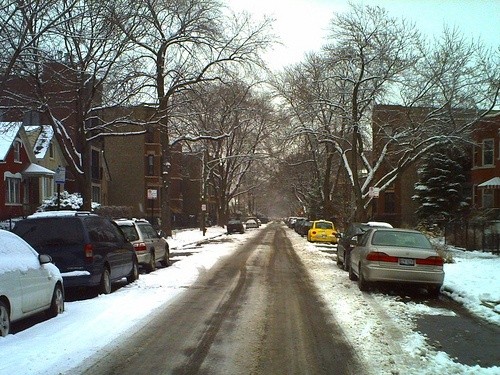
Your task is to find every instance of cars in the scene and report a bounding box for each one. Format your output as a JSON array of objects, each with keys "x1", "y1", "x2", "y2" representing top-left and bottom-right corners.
[
  {"x1": 334, "y1": 222, "x2": 396, "y2": 271},
  {"x1": 282, "y1": 217, "x2": 314, "y2": 237},
  {"x1": 348, "y1": 227, "x2": 445, "y2": 298},
  {"x1": 0, "y1": 229, "x2": 65, "y2": 337},
  {"x1": 112, "y1": 218, "x2": 170, "y2": 274},
  {"x1": 227, "y1": 220, "x2": 245, "y2": 235},
  {"x1": 245, "y1": 217, "x2": 271, "y2": 229},
  {"x1": 307, "y1": 219, "x2": 339, "y2": 245}
]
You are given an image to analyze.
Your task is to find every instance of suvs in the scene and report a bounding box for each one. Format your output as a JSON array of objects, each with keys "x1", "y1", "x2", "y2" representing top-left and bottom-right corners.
[{"x1": 10, "y1": 212, "x2": 139, "y2": 294}]
